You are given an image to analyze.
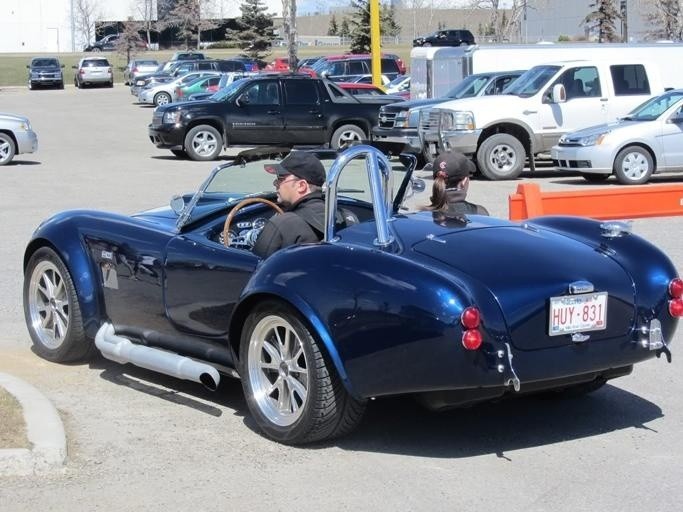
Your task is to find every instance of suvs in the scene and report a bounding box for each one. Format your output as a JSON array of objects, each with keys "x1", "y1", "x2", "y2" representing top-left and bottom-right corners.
[
  {"x1": 369, "y1": 69, "x2": 527, "y2": 169},
  {"x1": 410, "y1": 28, "x2": 479, "y2": 49},
  {"x1": 417, "y1": 59, "x2": 669, "y2": 182},
  {"x1": 26, "y1": 55, "x2": 66, "y2": 90}
]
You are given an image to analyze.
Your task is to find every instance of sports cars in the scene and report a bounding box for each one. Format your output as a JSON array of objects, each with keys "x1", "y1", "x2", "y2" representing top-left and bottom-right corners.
[{"x1": 21, "y1": 139, "x2": 683, "y2": 452}]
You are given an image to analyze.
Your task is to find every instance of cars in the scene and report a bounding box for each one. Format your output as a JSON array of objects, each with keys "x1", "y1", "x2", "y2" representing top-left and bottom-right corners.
[
  {"x1": 124, "y1": 39, "x2": 414, "y2": 107},
  {"x1": 82, "y1": 31, "x2": 152, "y2": 52},
  {"x1": 71, "y1": 57, "x2": 113, "y2": 89},
  {"x1": 550, "y1": 88, "x2": 683, "y2": 187},
  {"x1": 1, "y1": 112, "x2": 42, "y2": 169}
]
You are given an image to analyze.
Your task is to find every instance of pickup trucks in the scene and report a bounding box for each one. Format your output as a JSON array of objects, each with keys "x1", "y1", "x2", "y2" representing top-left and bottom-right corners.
[{"x1": 146, "y1": 73, "x2": 406, "y2": 161}]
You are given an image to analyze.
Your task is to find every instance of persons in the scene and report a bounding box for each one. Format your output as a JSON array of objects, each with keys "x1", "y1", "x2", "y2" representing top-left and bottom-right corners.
[
  {"x1": 412, "y1": 148, "x2": 489, "y2": 218},
  {"x1": 250, "y1": 150, "x2": 358, "y2": 260}
]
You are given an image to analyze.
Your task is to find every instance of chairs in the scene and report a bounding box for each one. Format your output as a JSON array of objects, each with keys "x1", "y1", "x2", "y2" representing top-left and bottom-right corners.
[{"x1": 570, "y1": 78, "x2": 599, "y2": 98}]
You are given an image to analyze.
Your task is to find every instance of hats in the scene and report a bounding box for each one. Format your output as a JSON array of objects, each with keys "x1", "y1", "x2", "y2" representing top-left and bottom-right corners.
[
  {"x1": 264, "y1": 151, "x2": 326, "y2": 186},
  {"x1": 433, "y1": 151, "x2": 476, "y2": 183}
]
